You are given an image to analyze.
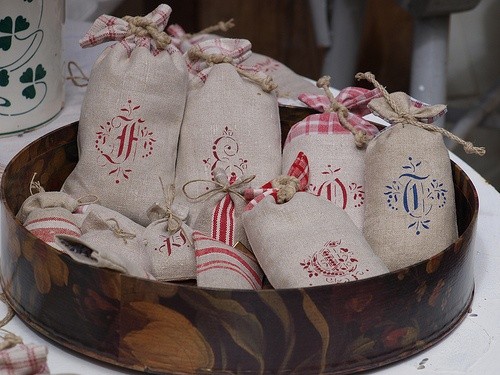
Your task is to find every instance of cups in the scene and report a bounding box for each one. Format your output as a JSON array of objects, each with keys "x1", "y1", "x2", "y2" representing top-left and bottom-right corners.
[{"x1": 1, "y1": 2, "x2": 66, "y2": 138}]
